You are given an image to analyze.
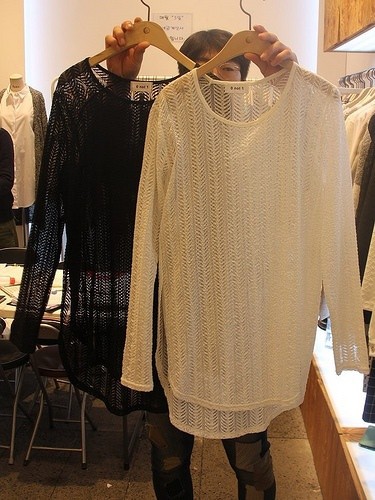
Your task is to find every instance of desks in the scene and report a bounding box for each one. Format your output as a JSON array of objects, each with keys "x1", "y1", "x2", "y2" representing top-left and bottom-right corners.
[{"x1": 0, "y1": 263, "x2": 148, "y2": 471}]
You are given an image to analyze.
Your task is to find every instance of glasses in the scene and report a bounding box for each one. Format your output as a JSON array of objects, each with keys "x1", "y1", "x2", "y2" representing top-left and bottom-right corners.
[{"x1": 198, "y1": 62, "x2": 240, "y2": 81}]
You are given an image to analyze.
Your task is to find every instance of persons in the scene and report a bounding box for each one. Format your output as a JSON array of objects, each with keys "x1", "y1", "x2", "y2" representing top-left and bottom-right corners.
[
  {"x1": 0, "y1": 129, "x2": 23, "y2": 248},
  {"x1": 103, "y1": 18, "x2": 297, "y2": 500}
]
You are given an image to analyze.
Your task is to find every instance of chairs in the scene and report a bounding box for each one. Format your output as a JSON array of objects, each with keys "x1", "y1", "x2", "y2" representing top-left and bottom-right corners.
[
  {"x1": 0, "y1": 316, "x2": 98, "y2": 471},
  {"x1": 0, "y1": 247, "x2": 28, "y2": 264}
]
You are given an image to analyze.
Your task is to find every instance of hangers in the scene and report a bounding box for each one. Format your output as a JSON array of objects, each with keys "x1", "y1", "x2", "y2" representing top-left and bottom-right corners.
[
  {"x1": 323, "y1": 68, "x2": 375, "y2": 126},
  {"x1": 58, "y1": 0, "x2": 228, "y2": 94},
  {"x1": 157, "y1": 0, "x2": 343, "y2": 100}
]
